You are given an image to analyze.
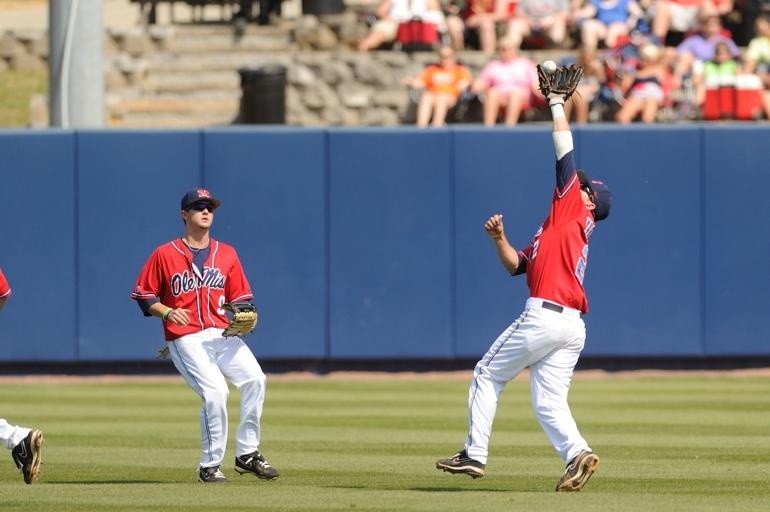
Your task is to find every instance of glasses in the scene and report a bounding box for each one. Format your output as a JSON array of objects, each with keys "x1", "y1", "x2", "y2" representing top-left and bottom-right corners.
[
  {"x1": 187, "y1": 202, "x2": 213, "y2": 212},
  {"x1": 580, "y1": 184, "x2": 593, "y2": 201}
]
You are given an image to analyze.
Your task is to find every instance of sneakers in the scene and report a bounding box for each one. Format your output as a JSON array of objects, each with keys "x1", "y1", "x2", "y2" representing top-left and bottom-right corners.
[
  {"x1": 199, "y1": 465, "x2": 227, "y2": 483},
  {"x1": 556, "y1": 451, "x2": 599, "y2": 491},
  {"x1": 235, "y1": 451, "x2": 280, "y2": 480},
  {"x1": 436, "y1": 451, "x2": 485, "y2": 479},
  {"x1": 11, "y1": 430, "x2": 43, "y2": 484}
]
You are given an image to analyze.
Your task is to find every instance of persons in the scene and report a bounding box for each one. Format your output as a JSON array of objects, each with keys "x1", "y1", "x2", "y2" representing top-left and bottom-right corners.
[
  {"x1": 407, "y1": 11, "x2": 769, "y2": 128},
  {"x1": 359, "y1": 0, "x2": 770, "y2": 54},
  {"x1": 0, "y1": 270, "x2": 44, "y2": 484},
  {"x1": 129, "y1": 187, "x2": 279, "y2": 483},
  {"x1": 436, "y1": 63, "x2": 613, "y2": 491}
]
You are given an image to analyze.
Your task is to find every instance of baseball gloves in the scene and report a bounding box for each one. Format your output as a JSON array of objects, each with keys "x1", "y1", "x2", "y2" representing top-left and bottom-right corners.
[
  {"x1": 220, "y1": 302, "x2": 258, "y2": 336},
  {"x1": 537, "y1": 64, "x2": 583, "y2": 100}
]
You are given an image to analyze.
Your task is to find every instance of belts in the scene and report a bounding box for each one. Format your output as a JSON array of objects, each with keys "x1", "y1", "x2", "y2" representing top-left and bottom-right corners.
[{"x1": 542, "y1": 301, "x2": 562, "y2": 314}]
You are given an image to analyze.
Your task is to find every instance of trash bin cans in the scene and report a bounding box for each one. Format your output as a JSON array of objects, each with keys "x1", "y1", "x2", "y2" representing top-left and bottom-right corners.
[{"x1": 236, "y1": 64, "x2": 287, "y2": 125}]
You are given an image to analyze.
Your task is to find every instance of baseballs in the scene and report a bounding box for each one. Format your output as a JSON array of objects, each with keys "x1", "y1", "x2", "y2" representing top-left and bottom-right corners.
[{"x1": 542, "y1": 59, "x2": 557, "y2": 72}]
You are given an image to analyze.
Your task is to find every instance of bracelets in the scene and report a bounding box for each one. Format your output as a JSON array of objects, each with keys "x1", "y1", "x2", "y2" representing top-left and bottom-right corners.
[{"x1": 162, "y1": 308, "x2": 172, "y2": 321}]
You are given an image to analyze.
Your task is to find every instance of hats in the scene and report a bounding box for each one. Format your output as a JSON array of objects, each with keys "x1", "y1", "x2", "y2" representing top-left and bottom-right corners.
[
  {"x1": 577, "y1": 169, "x2": 611, "y2": 220},
  {"x1": 181, "y1": 187, "x2": 220, "y2": 210}
]
